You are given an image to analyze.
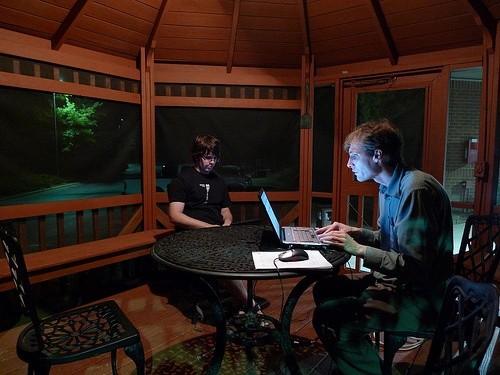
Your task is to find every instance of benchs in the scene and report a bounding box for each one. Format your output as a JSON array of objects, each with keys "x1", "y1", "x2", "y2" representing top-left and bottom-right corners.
[{"x1": 0, "y1": 193, "x2": 176, "y2": 293}]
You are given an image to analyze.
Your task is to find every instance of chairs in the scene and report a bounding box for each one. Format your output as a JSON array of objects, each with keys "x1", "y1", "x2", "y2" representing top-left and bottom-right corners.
[
  {"x1": 394, "y1": 275, "x2": 500, "y2": 375},
  {"x1": 368, "y1": 213, "x2": 500, "y2": 375},
  {"x1": 0, "y1": 229, "x2": 145, "y2": 375}
]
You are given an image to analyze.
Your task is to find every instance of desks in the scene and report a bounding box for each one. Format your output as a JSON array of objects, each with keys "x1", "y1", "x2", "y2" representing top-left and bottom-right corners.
[{"x1": 151, "y1": 224, "x2": 351, "y2": 375}]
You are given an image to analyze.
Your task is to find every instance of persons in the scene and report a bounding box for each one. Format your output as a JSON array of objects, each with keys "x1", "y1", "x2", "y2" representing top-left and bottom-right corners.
[
  {"x1": 168, "y1": 133, "x2": 270, "y2": 327},
  {"x1": 312, "y1": 120, "x2": 454, "y2": 375}
]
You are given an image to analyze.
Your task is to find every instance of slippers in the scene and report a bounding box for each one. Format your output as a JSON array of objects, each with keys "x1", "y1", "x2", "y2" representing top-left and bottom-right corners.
[{"x1": 236, "y1": 303, "x2": 271, "y2": 330}]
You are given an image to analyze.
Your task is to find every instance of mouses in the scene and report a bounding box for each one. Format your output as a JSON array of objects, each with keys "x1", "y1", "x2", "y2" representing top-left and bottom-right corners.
[{"x1": 279, "y1": 249, "x2": 309, "y2": 261}]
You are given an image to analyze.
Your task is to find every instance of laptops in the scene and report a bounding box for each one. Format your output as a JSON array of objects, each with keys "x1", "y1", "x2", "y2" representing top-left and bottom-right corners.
[{"x1": 257, "y1": 187, "x2": 340, "y2": 251}]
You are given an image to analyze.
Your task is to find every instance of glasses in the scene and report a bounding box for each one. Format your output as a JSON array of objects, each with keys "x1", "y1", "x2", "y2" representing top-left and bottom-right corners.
[
  {"x1": 348, "y1": 148, "x2": 368, "y2": 160},
  {"x1": 202, "y1": 156, "x2": 219, "y2": 163}
]
